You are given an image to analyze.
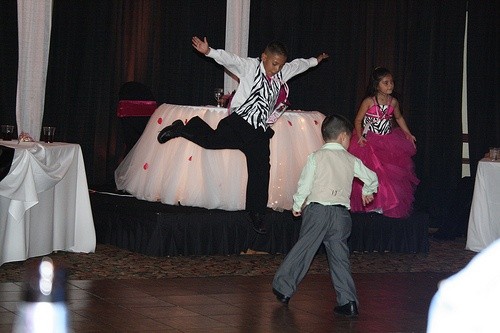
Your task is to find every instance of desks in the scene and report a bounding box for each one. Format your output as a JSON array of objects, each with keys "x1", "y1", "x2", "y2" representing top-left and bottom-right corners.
[
  {"x1": 1, "y1": 141, "x2": 97, "y2": 264},
  {"x1": 466, "y1": 161, "x2": 500, "y2": 253},
  {"x1": 115, "y1": 103, "x2": 327, "y2": 210}
]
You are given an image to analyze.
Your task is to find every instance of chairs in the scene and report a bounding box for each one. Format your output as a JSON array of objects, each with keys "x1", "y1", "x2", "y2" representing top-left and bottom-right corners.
[{"x1": 117, "y1": 100, "x2": 157, "y2": 160}]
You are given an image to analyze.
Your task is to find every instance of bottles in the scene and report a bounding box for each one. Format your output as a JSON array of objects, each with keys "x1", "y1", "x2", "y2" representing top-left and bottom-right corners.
[{"x1": 11, "y1": 258, "x2": 71, "y2": 332}]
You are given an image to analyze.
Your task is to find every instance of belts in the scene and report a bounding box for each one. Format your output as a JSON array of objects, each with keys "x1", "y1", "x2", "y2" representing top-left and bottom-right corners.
[{"x1": 310, "y1": 202, "x2": 346, "y2": 208}]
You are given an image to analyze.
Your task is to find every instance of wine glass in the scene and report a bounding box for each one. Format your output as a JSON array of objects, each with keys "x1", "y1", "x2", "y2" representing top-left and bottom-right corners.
[{"x1": 213, "y1": 88, "x2": 225, "y2": 108}]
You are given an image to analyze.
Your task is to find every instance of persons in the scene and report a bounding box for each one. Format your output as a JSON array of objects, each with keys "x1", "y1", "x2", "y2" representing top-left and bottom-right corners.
[
  {"x1": 272, "y1": 112, "x2": 380, "y2": 316},
  {"x1": 348, "y1": 65, "x2": 421, "y2": 220},
  {"x1": 158, "y1": 35, "x2": 330, "y2": 235}
]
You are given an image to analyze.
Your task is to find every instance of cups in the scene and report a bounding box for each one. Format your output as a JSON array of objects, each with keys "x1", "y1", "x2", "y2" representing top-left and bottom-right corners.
[
  {"x1": 2, "y1": 125, "x2": 14, "y2": 141},
  {"x1": 43, "y1": 127, "x2": 55, "y2": 143},
  {"x1": 489, "y1": 146, "x2": 499, "y2": 161}
]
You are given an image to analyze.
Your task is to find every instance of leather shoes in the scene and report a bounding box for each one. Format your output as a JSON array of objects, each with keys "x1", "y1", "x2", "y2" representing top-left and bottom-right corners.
[
  {"x1": 157, "y1": 119, "x2": 184, "y2": 144},
  {"x1": 334, "y1": 301, "x2": 358, "y2": 317},
  {"x1": 246, "y1": 212, "x2": 267, "y2": 234},
  {"x1": 272, "y1": 287, "x2": 290, "y2": 304}
]
